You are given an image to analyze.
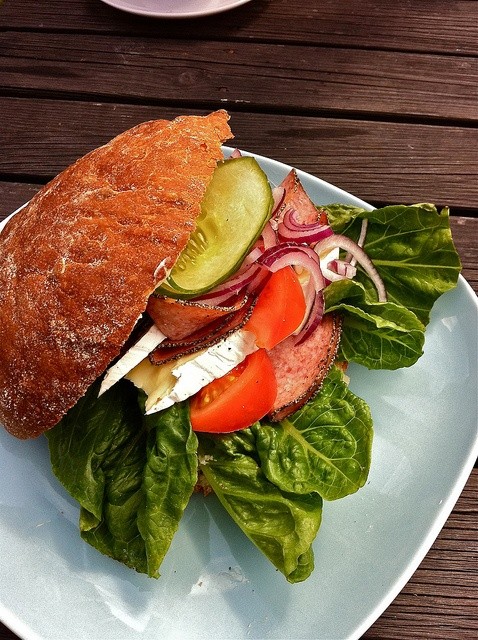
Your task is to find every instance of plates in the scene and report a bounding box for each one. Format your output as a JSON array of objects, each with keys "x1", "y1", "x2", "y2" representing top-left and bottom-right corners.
[
  {"x1": 0, "y1": 145, "x2": 477, "y2": 639},
  {"x1": 100, "y1": 0, "x2": 251, "y2": 17}
]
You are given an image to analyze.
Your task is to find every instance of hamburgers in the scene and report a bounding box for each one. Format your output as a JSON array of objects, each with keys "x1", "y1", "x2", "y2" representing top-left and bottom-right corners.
[{"x1": 0, "y1": 107, "x2": 462, "y2": 585}]
